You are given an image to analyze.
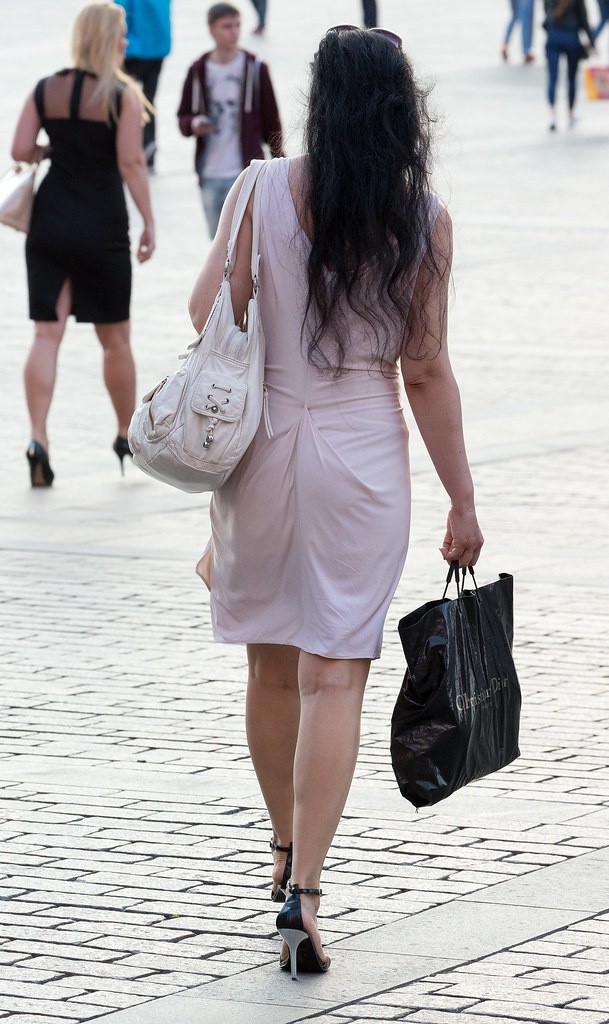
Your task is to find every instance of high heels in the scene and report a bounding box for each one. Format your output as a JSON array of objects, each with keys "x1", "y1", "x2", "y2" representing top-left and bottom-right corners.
[
  {"x1": 26, "y1": 441, "x2": 55, "y2": 487},
  {"x1": 276, "y1": 882, "x2": 331, "y2": 980},
  {"x1": 269, "y1": 837, "x2": 292, "y2": 902},
  {"x1": 114, "y1": 436, "x2": 133, "y2": 476}
]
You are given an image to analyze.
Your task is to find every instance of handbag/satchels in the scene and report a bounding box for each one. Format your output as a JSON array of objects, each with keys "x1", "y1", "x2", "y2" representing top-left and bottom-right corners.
[
  {"x1": 585, "y1": 66, "x2": 609, "y2": 101},
  {"x1": 390, "y1": 560, "x2": 522, "y2": 807},
  {"x1": 126, "y1": 159, "x2": 269, "y2": 493},
  {"x1": 0, "y1": 146, "x2": 44, "y2": 234}
]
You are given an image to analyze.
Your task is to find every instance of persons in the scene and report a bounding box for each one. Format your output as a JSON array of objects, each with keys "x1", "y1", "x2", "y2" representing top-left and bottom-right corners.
[
  {"x1": 502, "y1": 0, "x2": 535, "y2": 63},
  {"x1": 361, "y1": 0, "x2": 377, "y2": 29},
  {"x1": 187, "y1": 25, "x2": 483, "y2": 981},
  {"x1": 586, "y1": 0, "x2": 609, "y2": 48},
  {"x1": 251, "y1": 0, "x2": 268, "y2": 33},
  {"x1": 11, "y1": 2, "x2": 157, "y2": 489},
  {"x1": 178, "y1": 3, "x2": 283, "y2": 240},
  {"x1": 542, "y1": 0, "x2": 599, "y2": 131},
  {"x1": 113, "y1": 0, "x2": 171, "y2": 166}
]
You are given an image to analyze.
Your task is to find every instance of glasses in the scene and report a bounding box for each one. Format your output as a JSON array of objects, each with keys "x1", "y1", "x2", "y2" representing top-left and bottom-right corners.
[{"x1": 325, "y1": 24, "x2": 402, "y2": 53}]
